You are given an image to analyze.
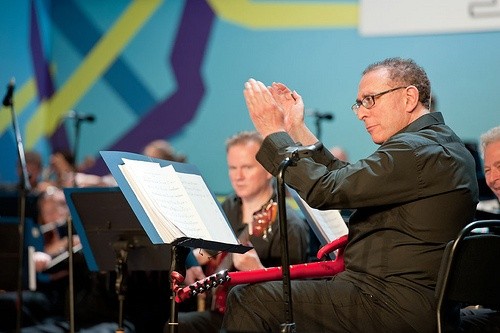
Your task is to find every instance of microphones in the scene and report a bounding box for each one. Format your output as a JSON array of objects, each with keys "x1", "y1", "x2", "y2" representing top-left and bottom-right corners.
[
  {"x1": 2, "y1": 78, "x2": 14, "y2": 106},
  {"x1": 68, "y1": 112, "x2": 94, "y2": 122},
  {"x1": 318, "y1": 114, "x2": 332, "y2": 119}
]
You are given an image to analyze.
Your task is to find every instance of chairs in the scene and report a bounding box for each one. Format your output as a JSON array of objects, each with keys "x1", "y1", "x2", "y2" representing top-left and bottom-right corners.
[{"x1": 434, "y1": 218, "x2": 500, "y2": 333}]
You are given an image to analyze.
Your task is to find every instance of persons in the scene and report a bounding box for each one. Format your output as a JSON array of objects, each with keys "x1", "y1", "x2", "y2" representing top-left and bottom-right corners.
[
  {"x1": 220, "y1": 56, "x2": 479, "y2": 333},
  {"x1": 479, "y1": 126, "x2": 500, "y2": 204},
  {"x1": 144, "y1": 139, "x2": 187, "y2": 163},
  {"x1": 165, "y1": 130, "x2": 310, "y2": 333},
  {"x1": 17, "y1": 151, "x2": 67, "y2": 254}
]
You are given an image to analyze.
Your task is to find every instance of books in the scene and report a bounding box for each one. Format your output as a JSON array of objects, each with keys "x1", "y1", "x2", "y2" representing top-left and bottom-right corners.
[
  {"x1": 286, "y1": 184, "x2": 349, "y2": 260},
  {"x1": 99, "y1": 150, "x2": 239, "y2": 244}
]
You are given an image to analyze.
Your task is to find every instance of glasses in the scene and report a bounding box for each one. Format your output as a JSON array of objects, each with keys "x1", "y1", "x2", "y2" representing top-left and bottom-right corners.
[{"x1": 351, "y1": 86, "x2": 408, "y2": 115}]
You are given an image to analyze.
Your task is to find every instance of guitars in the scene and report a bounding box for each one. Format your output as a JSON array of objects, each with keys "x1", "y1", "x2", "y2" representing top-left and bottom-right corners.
[
  {"x1": 171, "y1": 232, "x2": 349, "y2": 304},
  {"x1": 207, "y1": 200, "x2": 278, "y2": 313}
]
